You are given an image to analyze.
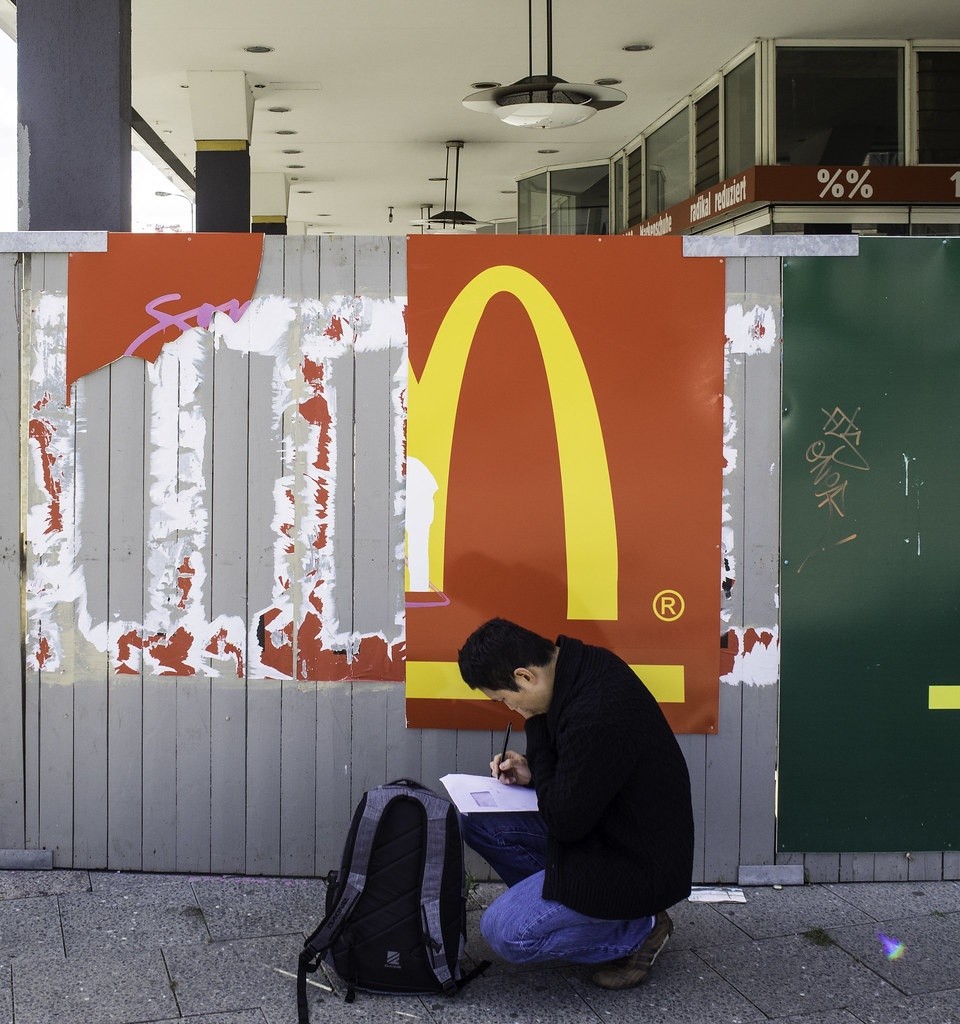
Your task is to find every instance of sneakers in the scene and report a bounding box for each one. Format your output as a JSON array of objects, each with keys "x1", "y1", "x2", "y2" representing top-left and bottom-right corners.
[{"x1": 594, "y1": 910, "x2": 674, "y2": 988}]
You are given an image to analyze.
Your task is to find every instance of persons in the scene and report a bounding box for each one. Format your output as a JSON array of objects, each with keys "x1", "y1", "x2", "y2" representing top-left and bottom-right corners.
[{"x1": 455, "y1": 617, "x2": 694, "y2": 987}]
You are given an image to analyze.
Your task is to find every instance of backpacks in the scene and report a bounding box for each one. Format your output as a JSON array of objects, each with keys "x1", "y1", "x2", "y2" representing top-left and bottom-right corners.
[{"x1": 297, "y1": 784, "x2": 491, "y2": 1024}]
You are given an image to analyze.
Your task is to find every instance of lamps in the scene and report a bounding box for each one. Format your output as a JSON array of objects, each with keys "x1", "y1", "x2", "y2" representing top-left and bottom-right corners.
[
  {"x1": 461, "y1": 0, "x2": 627, "y2": 129},
  {"x1": 408, "y1": 141, "x2": 495, "y2": 234}
]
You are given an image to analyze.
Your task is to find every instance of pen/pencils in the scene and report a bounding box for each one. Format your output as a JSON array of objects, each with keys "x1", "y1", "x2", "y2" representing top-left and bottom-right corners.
[{"x1": 496, "y1": 721, "x2": 512, "y2": 780}]
